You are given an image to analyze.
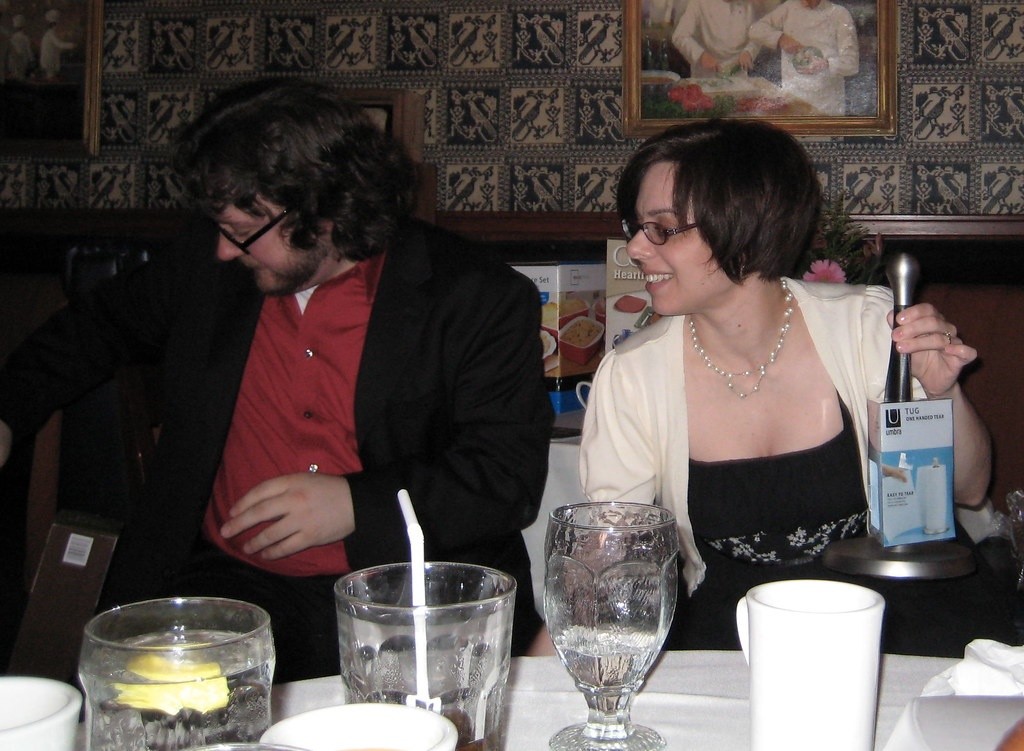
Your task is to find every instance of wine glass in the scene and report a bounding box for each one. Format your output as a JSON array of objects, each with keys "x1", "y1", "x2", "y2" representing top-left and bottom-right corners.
[{"x1": 541, "y1": 501, "x2": 679, "y2": 751}]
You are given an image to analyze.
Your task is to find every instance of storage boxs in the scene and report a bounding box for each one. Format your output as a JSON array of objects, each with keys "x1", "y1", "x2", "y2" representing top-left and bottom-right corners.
[
  {"x1": 508, "y1": 262, "x2": 606, "y2": 412},
  {"x1": 3, "y1": 507, "x2": 124, "y2": 683},
  {"x1": 604, "y1": 237, "x2": 661, "y2": 353}
]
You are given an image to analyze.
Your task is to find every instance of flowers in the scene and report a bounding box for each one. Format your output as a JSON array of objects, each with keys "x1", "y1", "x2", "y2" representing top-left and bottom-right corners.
[{"x1": 792, "y1": 188, "x2": 890, "y2": 285}]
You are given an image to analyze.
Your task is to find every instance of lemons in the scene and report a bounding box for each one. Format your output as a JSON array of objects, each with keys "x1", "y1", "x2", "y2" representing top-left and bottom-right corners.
[{"x1": 111, "y1": 654, "x2": 232, "y2": 715}]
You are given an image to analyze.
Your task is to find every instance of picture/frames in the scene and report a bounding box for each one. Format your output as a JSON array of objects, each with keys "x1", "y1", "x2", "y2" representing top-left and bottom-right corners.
[
  {"x1": 620, "y1": 0, "x2": 899, "y2": 139},
  {"x1": 0, "y1": 0, "x2": 105, "y2": 160}
]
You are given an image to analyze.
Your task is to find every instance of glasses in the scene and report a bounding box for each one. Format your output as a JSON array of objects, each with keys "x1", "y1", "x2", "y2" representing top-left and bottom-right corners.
[
  {"x1": 213, "y1": 203, "x2": 298, "y2": 256},
  {"x1": 620, "y1": 218, "x2": 698, "y2": 245}
]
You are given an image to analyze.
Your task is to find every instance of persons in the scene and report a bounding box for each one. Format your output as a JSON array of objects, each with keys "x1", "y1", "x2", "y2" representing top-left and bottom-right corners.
[
  {"x1": 533, "y1": 121, "x2": 1007, "y2": 655},
  {"x1": 1, "y1": 79, "x2": 557, "y2": 680}
]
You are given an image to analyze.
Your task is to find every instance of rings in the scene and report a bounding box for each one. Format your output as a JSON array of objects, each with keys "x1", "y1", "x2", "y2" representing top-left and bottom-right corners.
[{"x1": 944, "y1": 331, "x2": 952, "y2": 344}]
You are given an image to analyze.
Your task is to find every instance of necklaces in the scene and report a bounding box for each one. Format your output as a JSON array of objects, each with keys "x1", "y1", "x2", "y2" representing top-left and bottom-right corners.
[{"x1": 689, "y1": 280, "x2": 794, "y2": 398}]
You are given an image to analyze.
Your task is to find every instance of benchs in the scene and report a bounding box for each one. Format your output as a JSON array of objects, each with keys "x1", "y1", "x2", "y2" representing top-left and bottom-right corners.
[{"x1": 0, "y1": 271, "x2": 1024, "y2": 585}]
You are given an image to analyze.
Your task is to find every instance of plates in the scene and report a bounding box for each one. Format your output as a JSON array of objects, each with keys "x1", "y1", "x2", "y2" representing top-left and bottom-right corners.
[{"x1": 540, "y1": 330, "x2": 557, "y2": 359}]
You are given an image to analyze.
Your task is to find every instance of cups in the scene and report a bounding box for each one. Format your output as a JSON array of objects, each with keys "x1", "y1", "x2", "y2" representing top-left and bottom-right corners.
[
  {"x1": 916, "y1": 465, "x2": 949, "y2": 535},
  {"x1": 78, "y1": 596, "x2": 276, "y2": 751},
  {"x1": 736, "y1": 579, "x2": 886, "y2": 751},
  {"x1": 334, "y1": 562, "x2": 517, "y2": 751}
]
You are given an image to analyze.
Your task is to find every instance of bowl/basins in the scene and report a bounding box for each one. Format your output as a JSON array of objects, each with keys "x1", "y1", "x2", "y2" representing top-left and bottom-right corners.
[
  {"x1": 559, "y1": 299, "x2": 589, "y2": 330},
  {"x1": 257, "y1": 703, "x2": 459, "y2": 751},
  {"x1": 0, "y1": 677, "x2": 82, "y2": 751},
  {"x1": 557, "y1": 315, "x2": 605, "y2": 365}
]
